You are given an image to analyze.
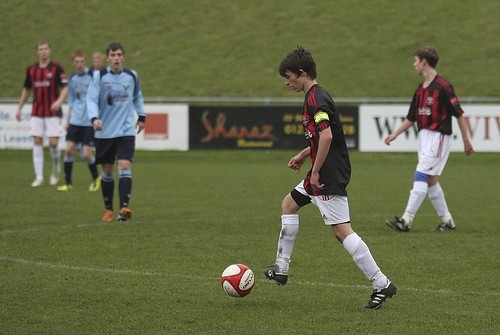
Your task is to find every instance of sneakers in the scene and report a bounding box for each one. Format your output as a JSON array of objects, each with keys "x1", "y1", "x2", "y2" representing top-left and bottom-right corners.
[
  {"x1": 386, "y1": 216, "x2": 412, "y2": 233},
  {"x1": 264, "y1": 267, "x2": 288, "y2": 286},
  {"x1": 102, "y1": 210, "x2": 114, "y2": 222},
  {"x1": 365, "y1": 283, "x2": 397, "y2": 309},
  {"x1": 49, "y1": 170, "x2": 60, "y2": 185},
  {"x1": 88, "y1": 175, "x2": 102, "y2": 191},
  {"x1": 57, "y1": 184, "x2": 74, "y2": 191},
  {"x1": 30, "y1": 178, "x2": 44, "y2": 187},
  {"x1": 118, "y1": 208, "x2": 133, "y2": 222},
  {"x1": 430, "y1": 221, "x2": 457, "y2": 233}
]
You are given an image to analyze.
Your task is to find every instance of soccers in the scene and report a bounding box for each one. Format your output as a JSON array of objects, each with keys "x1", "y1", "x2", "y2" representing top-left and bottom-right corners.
[{"x1": 221, "y1": 263, "x2": 255, "y2": 297}]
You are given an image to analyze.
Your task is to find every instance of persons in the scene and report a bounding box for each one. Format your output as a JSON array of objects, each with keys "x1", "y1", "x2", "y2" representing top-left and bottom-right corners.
[
  {"x1": 87, "y1": 42, "x2": 146, "y2": 222},
  {"x1": 58, "y1": 51, "x2": 107, "y2": 190},
  {"x1": 385, "y1": 48, "x2": 475, "y2": 233},
  {"x1": 263, "y1": 44, "x2": 398, "y2": 310},
  {"x1": 15, "y1": 41, "x2": 69, "y2": 188}
]
